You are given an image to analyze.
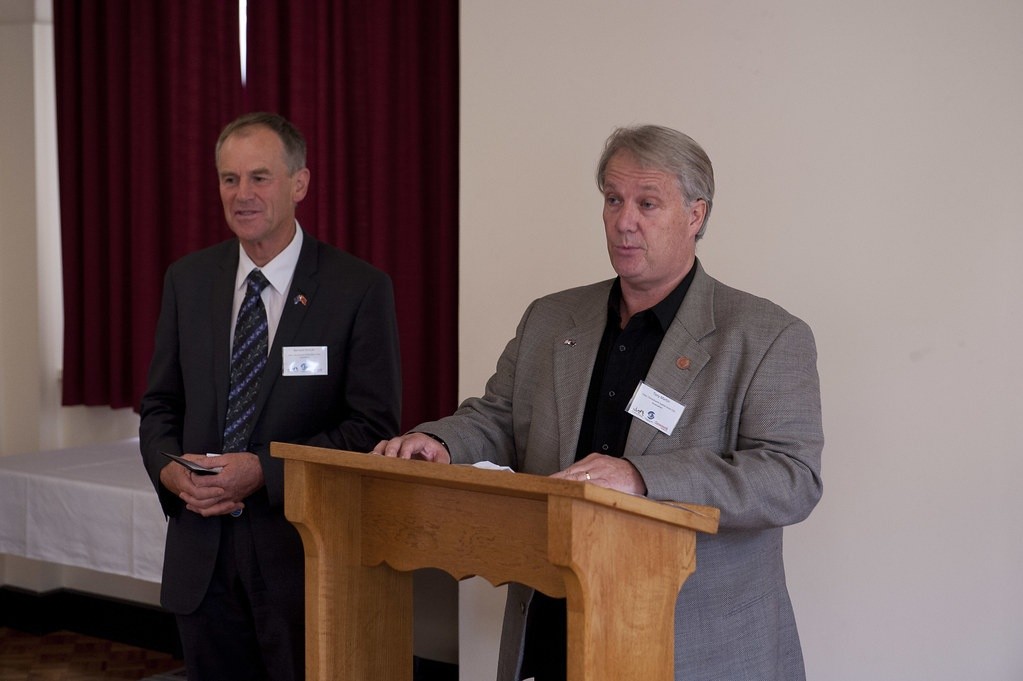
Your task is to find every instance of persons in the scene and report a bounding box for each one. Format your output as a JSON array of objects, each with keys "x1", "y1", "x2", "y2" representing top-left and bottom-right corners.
[
  {"x1": 371, "y1": 123, "x2": 826, "y2": 681},
  {"x1": 139, "y1": 113, "x2": 401, "y2": 681}
]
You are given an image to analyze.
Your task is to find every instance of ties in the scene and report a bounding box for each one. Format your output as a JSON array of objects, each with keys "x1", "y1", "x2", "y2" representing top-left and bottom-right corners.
[{"x1": 222, "y1": 266, "x2": 270, "y2": 454}]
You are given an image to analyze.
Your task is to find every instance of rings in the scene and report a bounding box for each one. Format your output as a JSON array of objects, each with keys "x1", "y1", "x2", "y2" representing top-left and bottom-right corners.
[{"x1": 585, "y1": 471, "x2": 590, "y2": 480}]
